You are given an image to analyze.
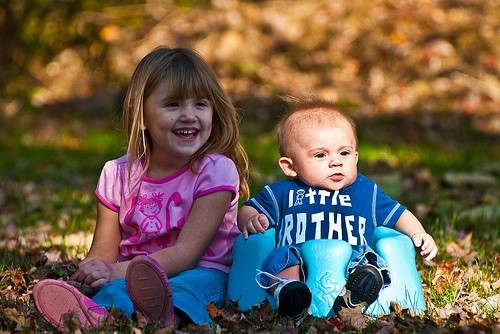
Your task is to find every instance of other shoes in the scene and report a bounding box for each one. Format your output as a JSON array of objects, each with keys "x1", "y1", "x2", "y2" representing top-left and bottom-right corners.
[
  {"x1": 331, "y1": 265, "x2": 384, "y2": 319},
  {"x1": 124, "y1": 254, "x2": 181, "y2": 333},
  {"x1": 253, "y1": 267, "x2": 312, "y2": 321},
  {"x1": 30, "y1": 278, "x2": 119, "y2": 333}
]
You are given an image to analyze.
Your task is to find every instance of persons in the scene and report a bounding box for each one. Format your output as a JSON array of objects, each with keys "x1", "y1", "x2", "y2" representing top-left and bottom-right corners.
[
  {"x1": 234, "y1": 95, "x2": 438, "y2": 334},
  {"x1": 34, "y1": 45, "x2": 254, "y2": 334}
]
